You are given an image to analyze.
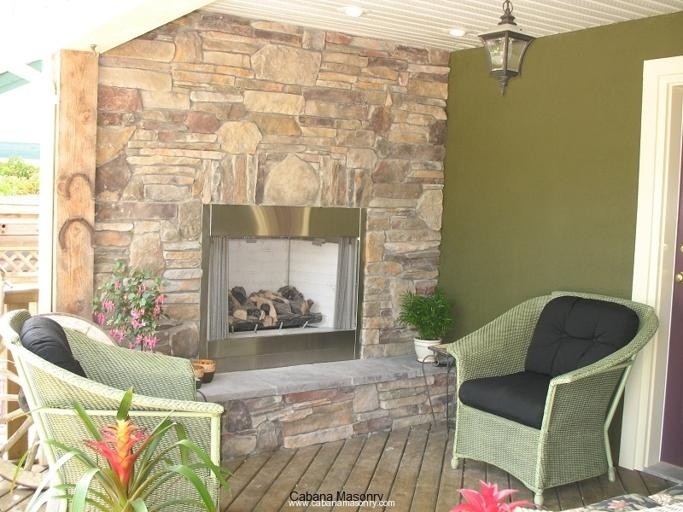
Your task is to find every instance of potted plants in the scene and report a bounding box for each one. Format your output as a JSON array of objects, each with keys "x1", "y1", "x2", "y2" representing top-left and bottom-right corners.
[{"x1": 396, "y1": 284, "x2": 451, "y2": 362}]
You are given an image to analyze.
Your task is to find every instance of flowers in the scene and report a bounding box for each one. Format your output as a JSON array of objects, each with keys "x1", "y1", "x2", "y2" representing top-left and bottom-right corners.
[
  {"x1": 25, "y1": 385, "x2": 241, "y2": 512},
  {"x1": 94, "y1": 260, "x2": 173, "y2": 351},
  {"x1": 450, "y1": 480, "x2": 536, "y2": 512}
]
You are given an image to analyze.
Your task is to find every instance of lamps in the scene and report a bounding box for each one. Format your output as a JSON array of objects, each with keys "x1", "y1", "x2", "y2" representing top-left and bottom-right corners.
[{"x1": 478, "y1": 0, "x2": 536, "y2": 97}]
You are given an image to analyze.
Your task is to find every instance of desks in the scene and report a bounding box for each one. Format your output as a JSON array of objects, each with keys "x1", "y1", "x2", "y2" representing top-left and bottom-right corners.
[{"x1": 422, "y1": 343, "x2": 456, "y2": 432}]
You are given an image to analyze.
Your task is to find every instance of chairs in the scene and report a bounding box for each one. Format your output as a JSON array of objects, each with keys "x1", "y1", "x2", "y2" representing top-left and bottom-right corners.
[
  {"x1": 0, "y1": 307, "x2": 225, "y2": 512},
  {"x1": 447, "y1": 291, "x2": 659, "y2": 510}
]
